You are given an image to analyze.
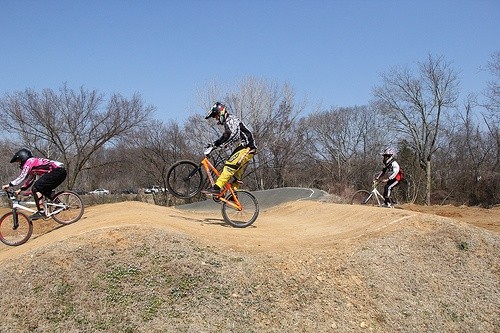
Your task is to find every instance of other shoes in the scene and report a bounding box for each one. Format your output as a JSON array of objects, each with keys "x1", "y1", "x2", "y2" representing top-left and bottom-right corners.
[
  {"x1": 229, "y1": 195, "x2": 238, "y2": 201},
  {"x1": 201, "y1": 187, "x2": 220, "y2": 194},
  {"x1": 382, "y1": 203, "x2": 388, "y2": 206}
]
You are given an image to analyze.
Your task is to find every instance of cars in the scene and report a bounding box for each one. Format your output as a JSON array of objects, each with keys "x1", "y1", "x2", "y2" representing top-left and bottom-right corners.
[
  {"x1": 71, "y1": 188, "x2": 88, "y2": 196},
  {"x1": 22, "y1": 187, "x2": 34, "y2": 197},
  {"x1": 0, "y1": 187, "x2": 17, "y2": 197},
  {"x1": 142, "y1": 185, "x2": 168, "y2": 194},
  {"x1": 88, "y1": 188, "x2": 110, "y2": 196}
]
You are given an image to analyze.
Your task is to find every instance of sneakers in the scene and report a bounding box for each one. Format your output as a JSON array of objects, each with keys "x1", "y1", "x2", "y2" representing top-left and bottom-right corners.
[
  {"x1": 28, "y1": 210, "x2": 46, "y2": 219},
  {"x1": 53, "y1": 203, "x2": 67, "y2": 214}
]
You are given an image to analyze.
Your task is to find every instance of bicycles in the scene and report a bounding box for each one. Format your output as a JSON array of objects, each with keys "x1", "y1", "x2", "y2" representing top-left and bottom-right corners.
[
  {"x1": 0, "y1": 184, "x2": 86, "y2": 247},
  {"x1": 351, "y1": 178, "x2": 396, "y2": 209},
  {"x1": 164, "y1": 143, "x2": 260, "y2": 228}
]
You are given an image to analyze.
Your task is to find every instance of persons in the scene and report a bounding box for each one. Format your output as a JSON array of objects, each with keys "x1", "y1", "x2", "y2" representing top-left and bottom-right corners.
[
  {"x1": 200, "y1": 101, "x2": 258, "y2": 203},
  {"x1": 2, "y1": 148, "x2": 67, "y2": 219},
  {"x1": 373, "y1": 149, "x2": 401, "y2": 207}
]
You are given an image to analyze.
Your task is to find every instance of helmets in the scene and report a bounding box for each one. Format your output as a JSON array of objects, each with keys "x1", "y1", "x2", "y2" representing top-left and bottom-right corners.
[
  {"x1": 380, "y1": 147, "x2": 395, "y2": 166},
  {"x1": 10, "y1": 149, "x2": 33, "y2": 166},
  {"x1": 205, "y1": 102, "x2": 229, "y2": 125}
]
